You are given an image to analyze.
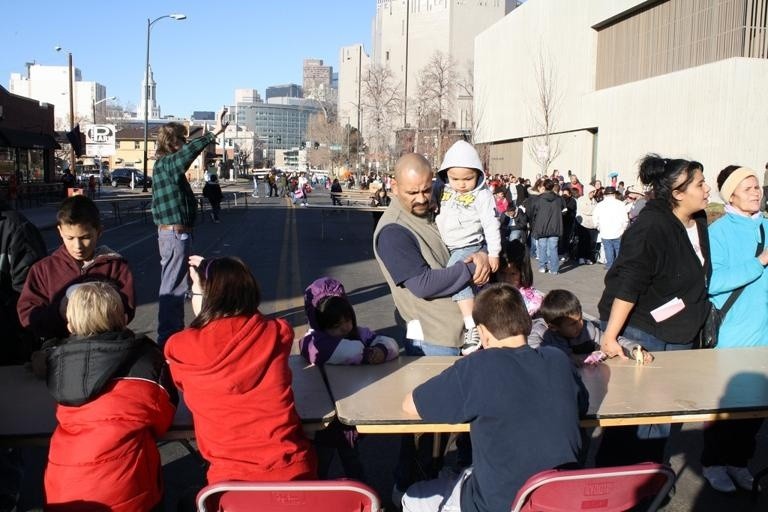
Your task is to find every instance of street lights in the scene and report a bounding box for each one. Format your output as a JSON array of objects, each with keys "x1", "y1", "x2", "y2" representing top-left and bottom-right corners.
[
  {"x1": 56, "y1": 47, "x2": 76, "y2": 181},
  {"x1": 143, "y1": 14, "x2": 187, "y2": 191}
]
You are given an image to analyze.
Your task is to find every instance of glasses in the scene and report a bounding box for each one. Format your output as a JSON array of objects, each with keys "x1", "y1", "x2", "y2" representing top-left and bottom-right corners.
[{"x1": 185, "y1": 290, "x2": 203, "y2": 300}]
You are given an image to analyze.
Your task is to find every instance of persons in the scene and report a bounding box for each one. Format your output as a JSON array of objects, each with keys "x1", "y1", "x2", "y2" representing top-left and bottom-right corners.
[
  {"x1": 402, "y1": 281, "x2": 590, "y2": 512},
  {"x1": 0, "y1": 201, "x2": 48, "y2": 367},
  {"x1": 299, "y1": 276, "x2": 401, "y2": 445},
  {"x1": 200, "y1": 174, "x2": 224, "y2": 224},
  {"x1": 15, "y1": 195, "x2": 136, "y2": 363},
  {"x1": 150, "y1": 107, "x2": 231, "y2": 350},
  {"x1": 34, "y1": 278, "x2": 178, "y2": 511},
  {"x1": 595, "y1": 151, "x2": 713, "y2": 439},
  {"x1": 88, "y1": 175, "x2": 97, "y2": 199},
  {"x1": 264, "y1": 167, "x2": 344, "y2": 207},
  {"x1": 698, "y1": 166, "x2": 768, "y2": 495},
  {"x1": 428, "y1": 140, "x2": 502, "y2": 357},
  {"x1": 527, "y1": 290, "x2": 653, "y2": 365},
  {"x1": 162, "y1": 254, "x2": 319, "y2": 511},
  {"x1": 8, "y1": 174, "x2": 23, "y2": 210},
  {"x1": 430, "y1": 167, "x2": 651, "y2": 275},
  {"x1": 347, "y1": 172, "x2": 397, "y2": 207},
  {"x1": 493, "y1": 239, "x2": 546, "y2": 315},
  {"x1": 61, "y1": 168, "x2": 76, "y2": 195},
  {"x1": 373, "y1": 152, "x2": 503, "y2": 359}
]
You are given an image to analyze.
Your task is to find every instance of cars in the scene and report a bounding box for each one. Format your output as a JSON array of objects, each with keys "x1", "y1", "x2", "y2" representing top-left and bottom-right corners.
[{"x1": 88, "y1": 169, "x2": 104, "y2": 183}]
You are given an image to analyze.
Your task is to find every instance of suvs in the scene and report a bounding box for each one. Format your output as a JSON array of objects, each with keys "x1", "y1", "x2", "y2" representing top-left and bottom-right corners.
[{"x1": 111, "y1": 168, "x2": 152, "y2": 188}]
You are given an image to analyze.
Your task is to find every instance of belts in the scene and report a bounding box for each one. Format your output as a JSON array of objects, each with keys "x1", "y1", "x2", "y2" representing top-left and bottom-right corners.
[{"x1": 160, "y1": 225, "x2": 192, "y2": 232}]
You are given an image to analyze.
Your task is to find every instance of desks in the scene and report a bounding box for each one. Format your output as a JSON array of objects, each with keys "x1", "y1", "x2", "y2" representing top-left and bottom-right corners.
[
  {"x1": 0, "y1": 354, "x2": 336, "y2": 462},
  {"x1": 323, "y1": 346, "x2": 768, "y2": 501}
]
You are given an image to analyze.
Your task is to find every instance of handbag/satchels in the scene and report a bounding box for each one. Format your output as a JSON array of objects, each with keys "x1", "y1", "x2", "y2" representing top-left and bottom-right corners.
[{"x1": 695, "y1": 302, "x2": 724, "y2": 348}]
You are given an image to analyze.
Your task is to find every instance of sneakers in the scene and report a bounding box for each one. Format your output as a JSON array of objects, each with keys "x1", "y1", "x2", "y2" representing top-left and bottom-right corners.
[
  {"x1": 462, "y1": 327, "x2": 481, "y2": 356},
  {"x1": 702, "y1": 467, "x2": 736, "y2": 491},
  {"x1": 539, "y1": 258, "x2": 592, "y2": 275},
  {"x1": 727, "y1": 466, "x2": 762, "y2": 491}
]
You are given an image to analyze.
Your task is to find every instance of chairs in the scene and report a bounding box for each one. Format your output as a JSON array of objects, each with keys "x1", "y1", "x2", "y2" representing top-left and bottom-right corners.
[
  {"x1": 195, "y1": 477, "x2": 382, "y2": 511},
  {"x1": 511, "y1": 462, "x2": 675, "y2": 512}
]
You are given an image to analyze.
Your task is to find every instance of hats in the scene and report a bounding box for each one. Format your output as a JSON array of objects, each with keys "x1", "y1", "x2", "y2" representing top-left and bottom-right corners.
[{"x1": 604, "y1": 188, "x2": 617, "y2": 194}]
